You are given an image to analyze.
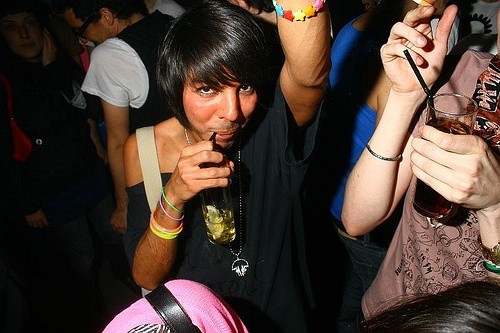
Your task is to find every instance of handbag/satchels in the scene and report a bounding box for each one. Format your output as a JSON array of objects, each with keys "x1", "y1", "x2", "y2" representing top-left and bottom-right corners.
[{"x1": 0, "y1": 72, "x2": 31, "y2": 162}]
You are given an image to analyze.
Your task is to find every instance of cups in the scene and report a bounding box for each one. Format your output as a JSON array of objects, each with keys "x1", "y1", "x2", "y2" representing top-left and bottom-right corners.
[
  {"x1": 413, "y1": 93, "x2": 480, "y2": 219},
  {"x1": 200, "y1": 155, "x2": 236, "y2": 248}
]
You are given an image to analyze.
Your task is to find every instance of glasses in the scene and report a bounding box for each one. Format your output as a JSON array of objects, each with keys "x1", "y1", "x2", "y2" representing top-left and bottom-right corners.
[{"x1": 72, "y1": 15, "x2": 100, "y2": 40}]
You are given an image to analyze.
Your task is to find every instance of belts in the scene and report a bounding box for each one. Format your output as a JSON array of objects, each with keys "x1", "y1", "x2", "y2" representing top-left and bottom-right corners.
[{"x1": 334, "y1": 218, "x2": 391, "y2": 242}]
